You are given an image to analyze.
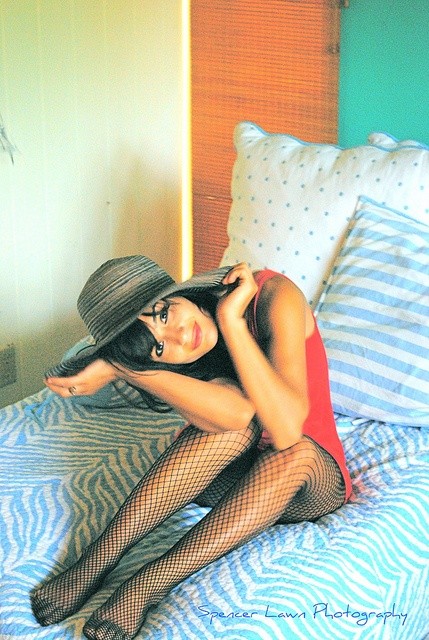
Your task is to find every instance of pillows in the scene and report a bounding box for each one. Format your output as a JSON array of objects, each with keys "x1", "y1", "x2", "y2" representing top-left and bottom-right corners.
[
  {"x1": 311, "y1": 191, "x2": 427, "y2": 431},
  {"x1": 217, "y1": 120, "x2": 428, "y2": 316},
  {"x1": 367, "y1": 130, "x2": 423, "y2": 152}
]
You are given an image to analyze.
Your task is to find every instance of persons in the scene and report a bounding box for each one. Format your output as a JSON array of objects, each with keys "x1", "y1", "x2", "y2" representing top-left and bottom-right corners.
[{"x1": 28, "y1": 254, "x2": 354, "y2": 640}]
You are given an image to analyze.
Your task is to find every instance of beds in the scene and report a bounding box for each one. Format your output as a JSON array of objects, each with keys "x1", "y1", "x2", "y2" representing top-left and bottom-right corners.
[{"x1": 0, "y1": 115, "x2": 427, "y2": 639}]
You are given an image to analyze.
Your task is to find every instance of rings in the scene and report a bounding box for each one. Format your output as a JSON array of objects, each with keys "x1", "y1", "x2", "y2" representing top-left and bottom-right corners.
[{"x1": 68, "y1": 386, "x2": 79, "y2": 397}]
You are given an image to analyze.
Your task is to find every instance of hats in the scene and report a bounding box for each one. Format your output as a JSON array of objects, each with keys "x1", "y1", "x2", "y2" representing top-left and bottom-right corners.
[{"x1": 43, "y1": 256, "x2": 237, "y2": 384}]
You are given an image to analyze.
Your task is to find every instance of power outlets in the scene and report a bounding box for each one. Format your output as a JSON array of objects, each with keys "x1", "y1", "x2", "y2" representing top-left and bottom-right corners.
[{"x1": 0, "y1": 346, "x2": 19, "y2": 391}]
